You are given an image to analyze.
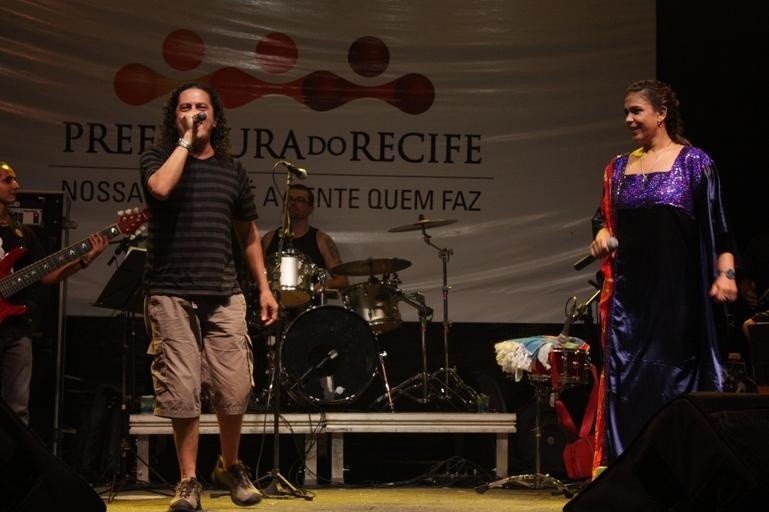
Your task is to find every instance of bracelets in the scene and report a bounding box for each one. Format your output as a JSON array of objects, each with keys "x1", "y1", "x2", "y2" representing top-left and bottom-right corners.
[
  {"x1": 176, "y1": 138, "x2": 193, "y2": 152},
  {"x1": 81, "y1": 256, "x2": 88, "y2": 269}
]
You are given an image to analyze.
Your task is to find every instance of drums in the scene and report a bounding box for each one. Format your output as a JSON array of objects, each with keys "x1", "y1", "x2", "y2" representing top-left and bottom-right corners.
[
  {"x1": 265, "y1": 250, "x2": 314, "y2": 309},
  {"x1": 344, "y1": 281, "x2": 401, "y2": 333},
  {"x1": 276, "y1": 305, "x2": 378, "y2": 407},
  {"x1": 527, "y1": 349, "x2": 590, "y2": 384}
]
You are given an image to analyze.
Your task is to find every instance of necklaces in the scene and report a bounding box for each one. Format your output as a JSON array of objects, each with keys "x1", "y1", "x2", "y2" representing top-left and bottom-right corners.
[{"x1": 639, "y1": 144, "x2": 674, "y2": 186}]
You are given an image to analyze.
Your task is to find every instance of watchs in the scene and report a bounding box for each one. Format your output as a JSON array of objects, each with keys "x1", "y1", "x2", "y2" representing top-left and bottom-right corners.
[{"x1": 714, "y1": 269, "x2": 736, "y2": 279}]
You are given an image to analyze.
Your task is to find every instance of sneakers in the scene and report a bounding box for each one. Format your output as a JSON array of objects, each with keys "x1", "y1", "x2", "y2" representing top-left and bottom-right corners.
[
  {"x1": 215, "y1": 456, "x2": 263, "y2": 505},
  {"x1": 170, "y1": 478, "x2": 202, "y2": 512}
]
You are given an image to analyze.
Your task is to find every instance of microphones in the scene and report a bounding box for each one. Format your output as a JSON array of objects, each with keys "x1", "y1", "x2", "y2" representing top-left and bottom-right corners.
[
  {"x1": 573, "y1": 237, "x2": 620, "y2": 272},
  {"x1": 282, "y1": 161, "x2": 308, "y2": 180},
  {"x1": 558, "y1": 304, "x2": 577, "y2": 345},
  {"x1": 310, "y1": 272, "x2": 328, "y2": 283},
  {"x1": 192, "y1": 110, "x2": 208, "y2": 125}
]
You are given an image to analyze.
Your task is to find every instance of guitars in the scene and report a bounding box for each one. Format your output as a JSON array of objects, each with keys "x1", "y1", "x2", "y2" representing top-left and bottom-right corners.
[{"x1": 1, "y1": 208, "x2": 149, "y2": 322}]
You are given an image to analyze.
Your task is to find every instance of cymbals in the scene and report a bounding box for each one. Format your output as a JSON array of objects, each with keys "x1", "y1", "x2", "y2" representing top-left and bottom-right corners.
[
  {"x1": 330, "y1": 259, "x2": 412, "y2": 276},
  {"x1": 388, "y1": 219, "x2": 458, "y2": 233}
]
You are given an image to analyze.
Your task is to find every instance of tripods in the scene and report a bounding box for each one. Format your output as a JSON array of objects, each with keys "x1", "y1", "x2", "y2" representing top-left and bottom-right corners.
[
  {"x1": 251, "y1": 317, "x2": 303, "y2": 499},
  {"x1": 472, "y1": 386, "x2": 574, "y2": 499},
  {"x1": 379, "y1": 249, "x2": 478, "y2": 412},
  {"x1": 101, "y1": 311, "x2": 176, "y2": 504},
  {"x1": 366, "y1": 291, "x2": 475, "y2": 412}
]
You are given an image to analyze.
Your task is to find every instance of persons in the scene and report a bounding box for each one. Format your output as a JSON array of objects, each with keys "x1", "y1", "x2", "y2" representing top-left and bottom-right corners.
[
  {"x1": 139, "y1": 79, "x2": 280, "y2": 511},
  {"x1": 261, "y1": 185, "x2": 350, "y2": 292},
  {"x1": 574, "y1": 79, "x2": 737, "y2": 460},
  {"x1": 0, "y1": 159, "x2": 109, "y2": 421}
]
